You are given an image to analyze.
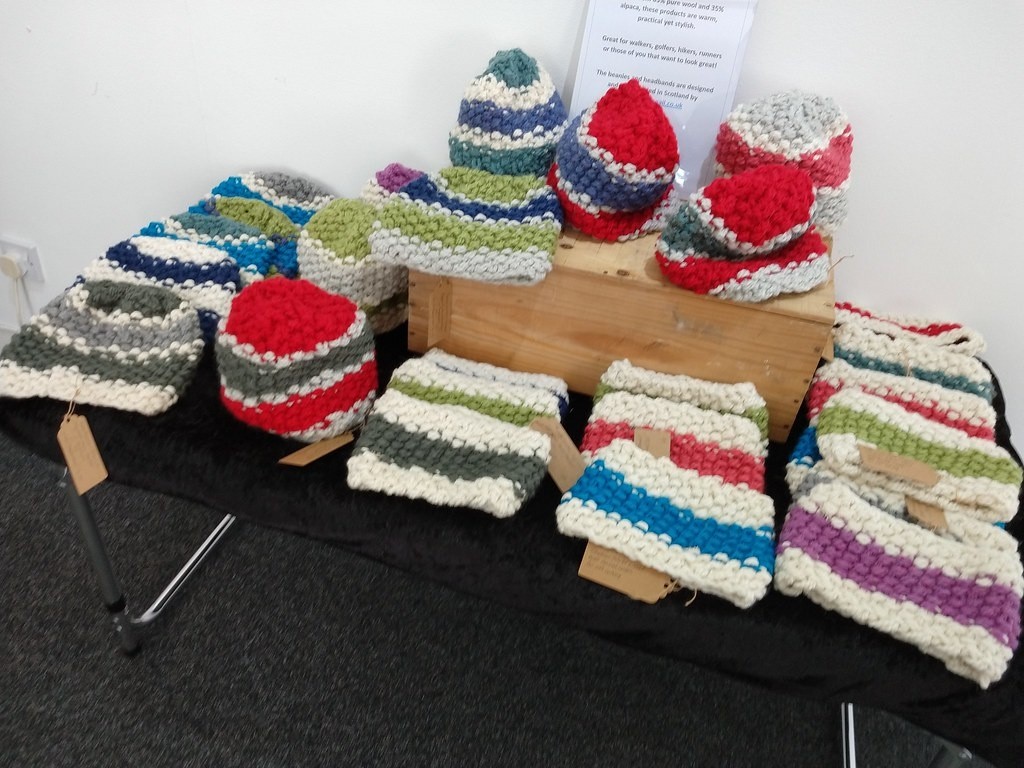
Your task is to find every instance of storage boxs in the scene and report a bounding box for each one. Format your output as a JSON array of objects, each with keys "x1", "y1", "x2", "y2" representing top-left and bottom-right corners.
[{"x1": 404, "y1": 233, "x2": 839, "y2": 450}]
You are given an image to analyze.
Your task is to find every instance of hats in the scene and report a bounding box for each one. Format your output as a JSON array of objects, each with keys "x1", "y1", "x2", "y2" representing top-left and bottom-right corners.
[
  {"x1": 652, "y1": 163, "x2": 832, "y2": 304},
  {"x1": 366, "y1": 168, "x2": 562, "y2": 290},
  {"x1": 89, "y1": 173, "x2": 347, "y2": 327},
  {"x1": 543, "y1": 79, "x2": 680, "y2": 241},
  {"x1": 217, "y1": 279, "x2": 377, "y2": 444},
  {"x1": 0, "y1": 277, "x2": 202, "y2": 416},
  {"x1": 448, "y1": 47, "x2": 566, "y2": 176},
  {"x1": 717, "y1": 90, "x2": 854, "y2": 233},
  {"x1": 298, "y1": 198, "x2": 407, "y2": 306}
]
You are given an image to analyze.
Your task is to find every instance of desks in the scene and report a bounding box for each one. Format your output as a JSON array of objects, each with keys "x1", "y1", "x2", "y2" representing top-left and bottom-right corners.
[{"x1": 0, "y1": 170, "x2": 1024, "y2": 768}]
